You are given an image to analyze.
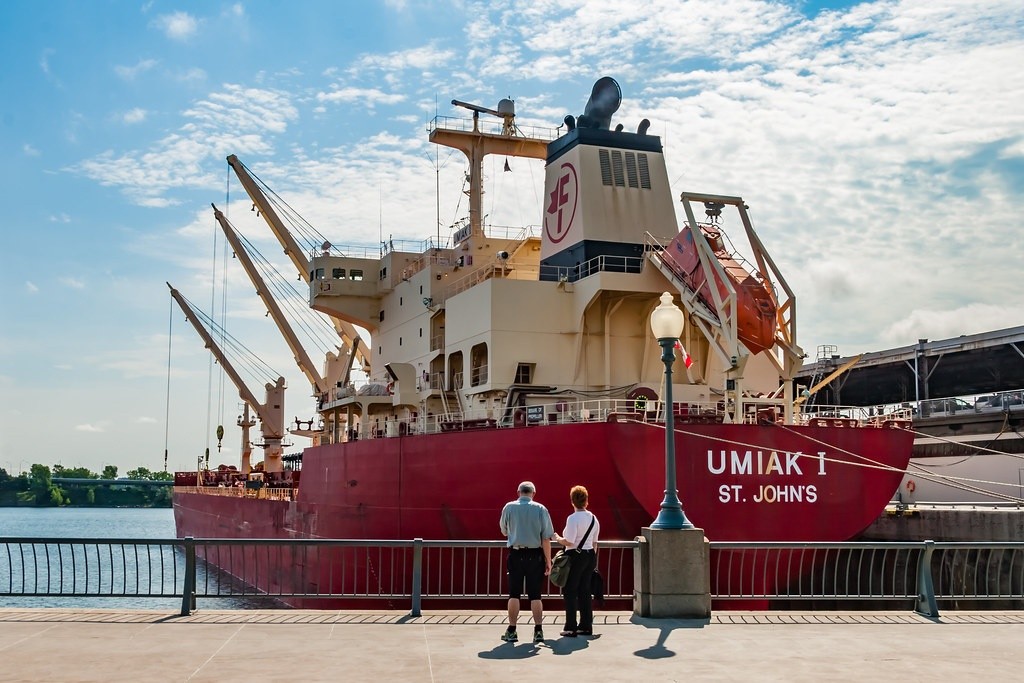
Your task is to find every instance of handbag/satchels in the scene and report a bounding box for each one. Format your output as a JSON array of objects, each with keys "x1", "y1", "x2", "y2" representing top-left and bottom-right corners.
[{"x1": 549, "y1": 549, "x2": 571, "y2": 589}]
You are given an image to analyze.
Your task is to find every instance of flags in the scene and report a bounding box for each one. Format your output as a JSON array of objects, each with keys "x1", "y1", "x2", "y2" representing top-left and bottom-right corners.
[{"x1": 674, "y1": 339, "x2": 693, "y2": 369}]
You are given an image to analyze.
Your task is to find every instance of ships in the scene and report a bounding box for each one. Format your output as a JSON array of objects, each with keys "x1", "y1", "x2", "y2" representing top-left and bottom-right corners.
[{"x1": 166, "y1": 76, "x2": 915, "y2": 610}]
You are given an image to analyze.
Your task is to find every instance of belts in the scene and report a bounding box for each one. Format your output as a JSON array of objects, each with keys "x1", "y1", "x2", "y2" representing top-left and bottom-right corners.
[{"x1": 512, "y1": 544, "x2": 539, "y2": 550}]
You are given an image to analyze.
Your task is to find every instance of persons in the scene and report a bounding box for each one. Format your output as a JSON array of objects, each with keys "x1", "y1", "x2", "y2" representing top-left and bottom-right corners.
[
  {"x1": 551, "y1": 487, "x2": 599, "y2": 636},
  {"x1": 500, "y1": 481, "x2": 554, "y2": 642}
]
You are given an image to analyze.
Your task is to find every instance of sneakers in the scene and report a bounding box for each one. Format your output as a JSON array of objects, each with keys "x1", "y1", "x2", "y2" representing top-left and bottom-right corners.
[
  {"x1": 501, "y1": 629, "x2": 518, "y2": 642},
  {"x1": 534, "y1": 631, "x2": 544, "y2": 642}
]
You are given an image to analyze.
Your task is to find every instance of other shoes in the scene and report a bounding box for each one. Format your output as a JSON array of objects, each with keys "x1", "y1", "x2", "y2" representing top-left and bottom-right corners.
[
  {"x1": 576, "y1": 626, "x2": 592, "y2": 635},
  {"x1": 559, "y1": 630, "x2": 576, "y2": 637}
]
